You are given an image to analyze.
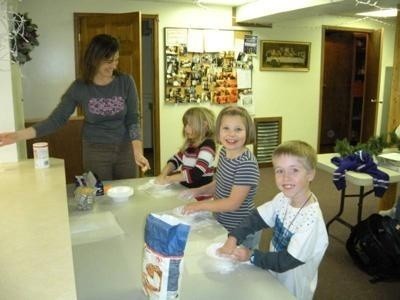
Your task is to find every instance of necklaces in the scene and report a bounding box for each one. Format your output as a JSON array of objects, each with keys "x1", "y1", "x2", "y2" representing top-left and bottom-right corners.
[{"x1": 274, "y1": 192, "x2": 313, "y2": 252}]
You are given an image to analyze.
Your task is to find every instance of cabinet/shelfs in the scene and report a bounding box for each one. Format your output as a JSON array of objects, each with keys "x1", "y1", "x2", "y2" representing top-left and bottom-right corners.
[{"x1": 348, "y1": 32, "x2": 369, "y2": 143}]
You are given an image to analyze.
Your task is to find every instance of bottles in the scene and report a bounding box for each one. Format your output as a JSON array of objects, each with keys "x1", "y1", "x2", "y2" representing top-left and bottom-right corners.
[{"x1": 31, "y1": 141, "x2": 49, "y2": 169}]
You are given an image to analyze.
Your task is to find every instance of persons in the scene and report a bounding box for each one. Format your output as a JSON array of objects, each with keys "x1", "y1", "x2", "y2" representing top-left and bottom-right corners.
[
  {"x1": 216, "y1": 139, "x2": 330, "y2": 300},
  {"x1": 178, "y1": 105, "x2": 260, "y2": 252},
  {"x1": 154, "y1": 106, "x2": 218, "y2": 217},
  {"x1": 0, "y1": 33, "x2": 151, "y2": 180},
  {"x1": 270, "y1": 46, "x2": 299, "y2": 67}
]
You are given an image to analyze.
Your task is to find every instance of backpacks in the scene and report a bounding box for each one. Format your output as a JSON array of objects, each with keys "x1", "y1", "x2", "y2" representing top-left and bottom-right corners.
[{"x1": 346, "y1": 211, "x2": 400, "y2": 284}]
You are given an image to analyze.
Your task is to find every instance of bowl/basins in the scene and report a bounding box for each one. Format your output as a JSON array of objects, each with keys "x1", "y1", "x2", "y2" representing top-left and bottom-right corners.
[
  {"x1": 107, "y1": 186, "x2": 134, "y2": 204},
  {"x1": 150, "y1": 178, "x2": 171, "y2": 191}
]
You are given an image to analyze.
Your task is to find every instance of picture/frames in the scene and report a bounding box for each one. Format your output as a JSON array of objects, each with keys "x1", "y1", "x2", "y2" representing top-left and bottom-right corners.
[{"x1": 260, "y1": 39, "x2": 311, "y2": 72}]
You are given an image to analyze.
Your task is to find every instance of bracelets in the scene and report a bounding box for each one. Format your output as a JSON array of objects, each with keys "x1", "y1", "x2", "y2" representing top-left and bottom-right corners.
[{"x1": 250, "y1": 251, "x2": 254, "y2": 264}]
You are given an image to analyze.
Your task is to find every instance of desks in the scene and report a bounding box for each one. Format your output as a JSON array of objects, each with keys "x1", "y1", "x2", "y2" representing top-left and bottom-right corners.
[
  {"x1": 315, "y1": 147, "x2": 400, "y2": 234},
  {"x1": 0, "y1": 157, "x2": 77, "y2": 300},
  {"x1": 67, "y1": 171, "x2": 298, "y2": 300}
]
